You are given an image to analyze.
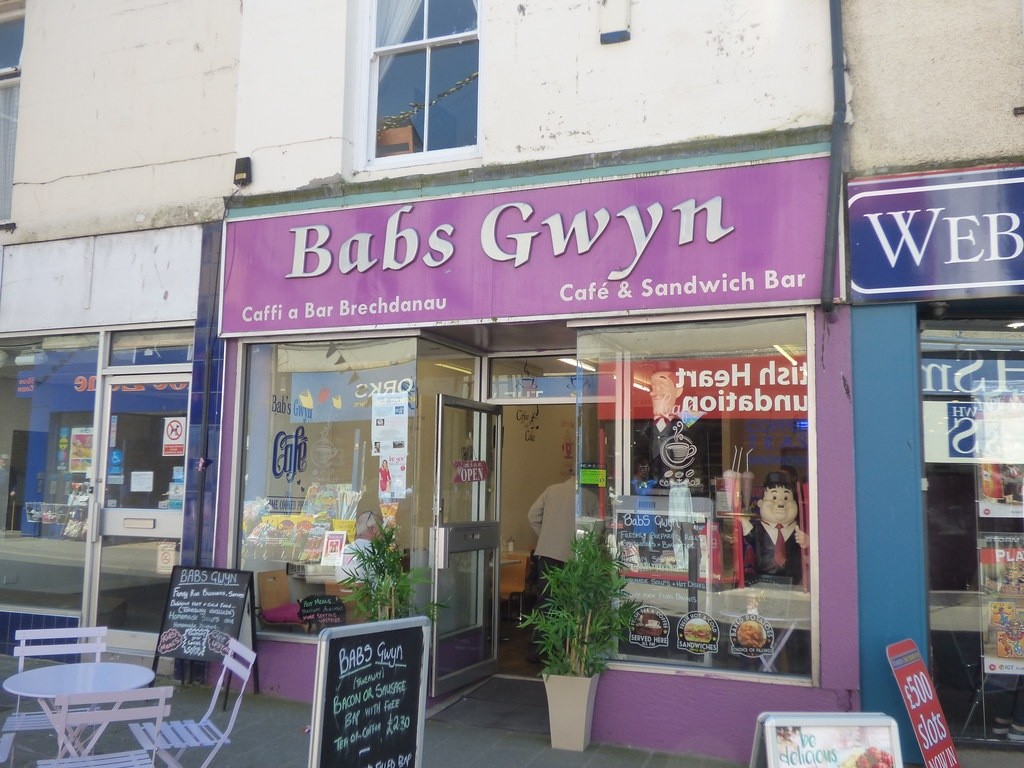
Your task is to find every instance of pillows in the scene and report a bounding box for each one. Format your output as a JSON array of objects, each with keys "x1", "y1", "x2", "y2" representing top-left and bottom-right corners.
[{"x1": 263, "y1": 602, "x2": 308, "y2": 625}]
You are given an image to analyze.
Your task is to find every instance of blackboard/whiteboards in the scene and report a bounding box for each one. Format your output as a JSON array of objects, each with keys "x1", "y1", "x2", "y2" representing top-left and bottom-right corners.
[
  {"x1": 296, "y1": 594, "x2": 347, "y2": 626},
  {"x1": 306, "y1": 616, "x2": 433, "y2": 767},
  {"x1": 154, "y1": 565, "x2": 259, "y2": 663}
]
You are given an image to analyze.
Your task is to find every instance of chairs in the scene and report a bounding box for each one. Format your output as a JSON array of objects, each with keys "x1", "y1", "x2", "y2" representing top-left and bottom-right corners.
[
  {"x1": 0, "y1": 626, "x2": 257, "y2": 768},
  {"x1": 260, "y1": 571, "x2": 317, "y2": 634}
]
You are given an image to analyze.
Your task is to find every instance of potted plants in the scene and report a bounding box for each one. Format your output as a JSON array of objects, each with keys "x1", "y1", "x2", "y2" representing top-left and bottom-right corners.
[{"x1": 521, "y1": 518, "x2": 643, "y2": 750}]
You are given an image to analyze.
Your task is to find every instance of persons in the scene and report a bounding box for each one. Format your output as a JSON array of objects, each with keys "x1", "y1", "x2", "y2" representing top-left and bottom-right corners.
[
  {"x1": 633, "y1": 455, "x2": 654, "y2": 482},
  {"x1": 525, "y1": 459, "x2": 599, "y2": 665},
  {"x1": 0, "y1": 453, "x2": 17, "y2": 532},
  {"x1": 779, "y1": 465, "x2": 803, "y2": 494},
  {"x1": 378, "y1": 460, "x2": 392, "y2": 492},
  {"x1": 334, "y1": 510, "x2": 400, "y2": 622},
  {"x1": 738, "y1": 472, "x2": 809, "y2": 586},
  {"x1": 957, "y1": 571, "x2": 1024, "y2": 741}
]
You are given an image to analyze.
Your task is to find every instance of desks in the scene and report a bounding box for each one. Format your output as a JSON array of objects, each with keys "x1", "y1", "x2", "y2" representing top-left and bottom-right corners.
[{"x1": 2, "y1": 662, "x2": 156, "y2": 757}]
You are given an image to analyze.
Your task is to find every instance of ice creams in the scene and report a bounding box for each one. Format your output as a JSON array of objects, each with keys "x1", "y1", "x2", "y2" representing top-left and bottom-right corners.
[{"x1": 722, "y1": 469, "x2": 755, "y2": 511}]
